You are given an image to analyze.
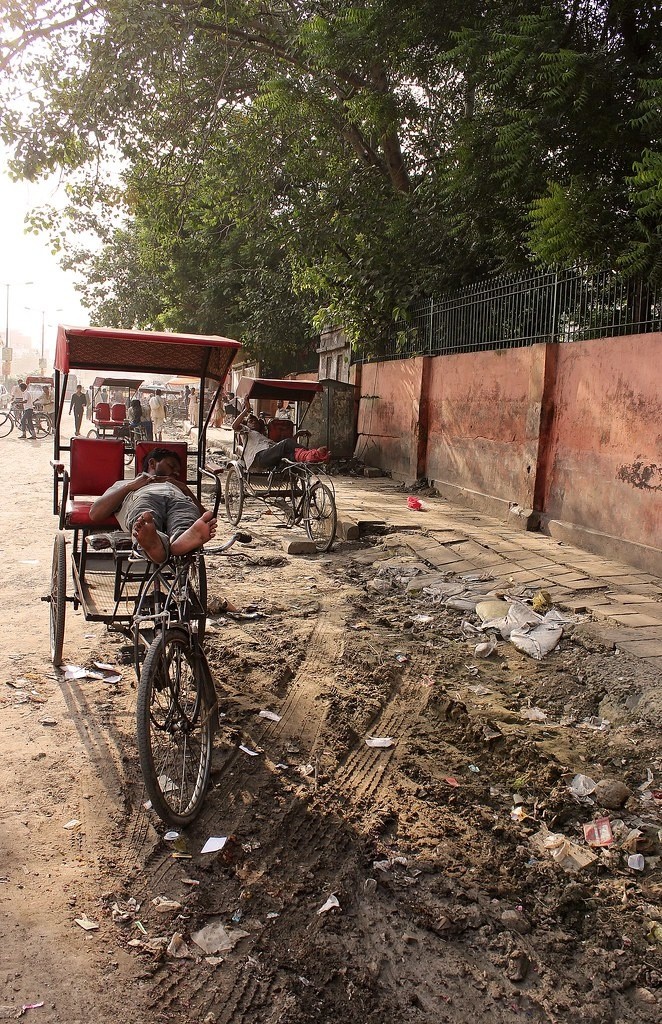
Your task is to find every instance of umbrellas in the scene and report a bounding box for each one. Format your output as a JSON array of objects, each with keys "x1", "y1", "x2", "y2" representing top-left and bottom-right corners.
[{"x1": 164, "y1": 375, "x2": 200, "y2": 393}]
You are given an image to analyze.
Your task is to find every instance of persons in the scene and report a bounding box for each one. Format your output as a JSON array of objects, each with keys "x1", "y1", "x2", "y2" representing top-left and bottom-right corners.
[
  {"x1": 231, "y1": 393, "x2": 332, "y2": 471},
  {"x1": 82, "y1": 385, "x2": 244, "y2": 431},
  {"x1": 89, "y1": 447, "x2": 218, "y2": 564},
  {"x1": 149, "y1": 390, "x2": 168, "y2": 443},
  {"x1": 33, "y1": 385, "x2": 55, "y2": 434},
  {"x1": 68, "y1": 385, "x2": 87, "y2": 436},
  {"x1": 274, "y1": 398, "x2": 291, "y2": 419},
  {"x1": 126, "y1": 399, "x2": 154, "y2": 445},
  {"x1": 12, "y1": 381, "x2": 36, "y2": 439},
  {"x1": 9, "y1": 379, "x2": 25, "y2": 420}
]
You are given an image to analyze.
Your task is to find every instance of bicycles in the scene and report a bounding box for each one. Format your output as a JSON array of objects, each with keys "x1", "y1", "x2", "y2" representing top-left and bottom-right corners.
[{"x1": 0, "y1": 395, "x2": 51, "y2": 439}]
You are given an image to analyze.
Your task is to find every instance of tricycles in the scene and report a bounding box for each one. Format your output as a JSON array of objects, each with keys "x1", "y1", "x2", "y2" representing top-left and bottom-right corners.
[
  {"x1": 23, "y1": 376, "x2": 55, "y2": 434},
  {"x1": 41, "y1": 324, "x2": 243, "y2": 828},
  {"x1": 88, "y1": 377, "x2": 154, "y2": 465},
  {"x1": 224, "y1": 377, "x2": 337, "y2": 553}
]
